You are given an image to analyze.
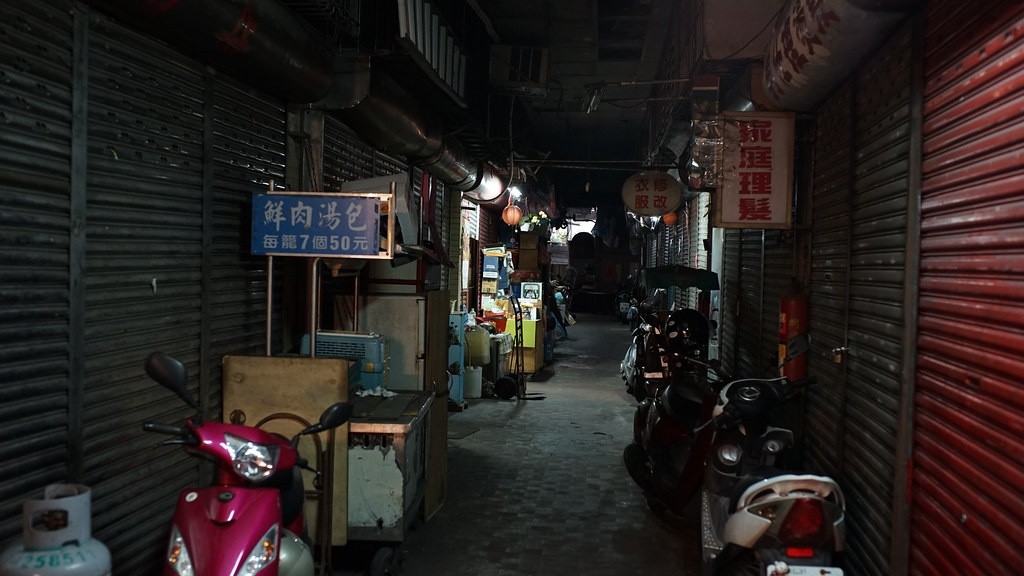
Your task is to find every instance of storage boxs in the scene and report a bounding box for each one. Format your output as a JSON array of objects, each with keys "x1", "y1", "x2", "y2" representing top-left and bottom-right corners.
[
  {"x1": 480, "y1": 255, "x2": 510, "y2": 295},
  {"x1": 518, "y1": 234, "x2": 540, "y2": 271}
]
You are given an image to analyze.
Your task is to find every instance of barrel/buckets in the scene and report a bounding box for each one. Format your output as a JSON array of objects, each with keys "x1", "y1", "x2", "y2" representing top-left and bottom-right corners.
[
  {"x1": 464, "y1": 326, "x2": 490, "y2": 366},
  {"x1": 464, "y1": 367, "x2": 482, "y2": 398}
]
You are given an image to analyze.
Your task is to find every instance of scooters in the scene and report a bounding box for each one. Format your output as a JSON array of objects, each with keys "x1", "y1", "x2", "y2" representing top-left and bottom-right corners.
[
  {"x1": 138, "y1": 349, "x2": 356, "y2": 576},
  {"x1": 620, "y1": 287, "x2": 856, "y2": 576}
]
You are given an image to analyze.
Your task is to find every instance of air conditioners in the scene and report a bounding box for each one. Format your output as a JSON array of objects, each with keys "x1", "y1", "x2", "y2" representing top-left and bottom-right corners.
[{"x1": 487, "y1": 44, "x2": 553, "y2": 95}]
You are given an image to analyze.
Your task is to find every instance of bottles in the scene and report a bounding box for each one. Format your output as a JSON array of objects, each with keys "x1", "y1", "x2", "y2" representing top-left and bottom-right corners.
[{"x1": 469, "y1": 308, "x2": 477, "y2": 325}]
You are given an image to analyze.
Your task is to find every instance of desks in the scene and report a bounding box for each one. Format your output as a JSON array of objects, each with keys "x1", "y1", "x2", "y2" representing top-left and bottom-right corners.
[
  {"x1": 483, "y1": 333, "x2": 513, "y2": 382},
  {"x1": 346, "y1": 389, "x2": 436, "y2": 544}
]
